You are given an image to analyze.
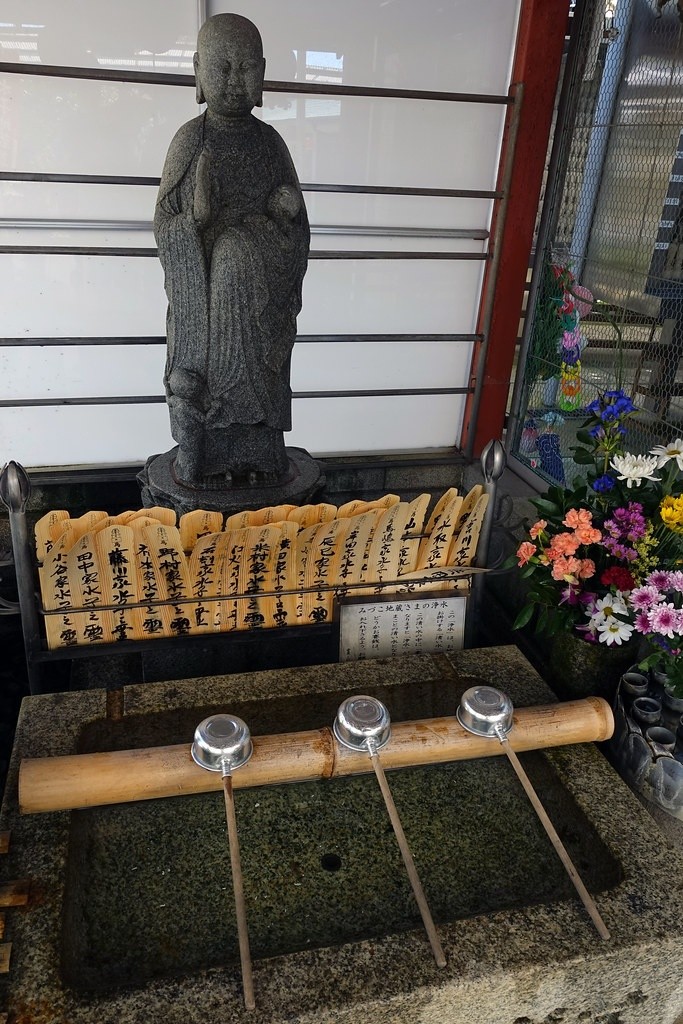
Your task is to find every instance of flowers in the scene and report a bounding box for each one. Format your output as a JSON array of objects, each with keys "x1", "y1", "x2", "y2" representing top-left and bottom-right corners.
[{"x1": 497, "y1": 389, "x2": 683, "y2": 700}]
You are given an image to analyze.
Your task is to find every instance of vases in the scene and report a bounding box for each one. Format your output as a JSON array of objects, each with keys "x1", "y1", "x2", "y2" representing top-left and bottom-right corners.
[{"x1": 546, "y1": 629, "x2": 641, "y2": 701}]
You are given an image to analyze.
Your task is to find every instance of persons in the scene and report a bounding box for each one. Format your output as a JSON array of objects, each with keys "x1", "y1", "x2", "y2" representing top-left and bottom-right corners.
[{"x1": 151, "y1": 14, "x2": 311, "y2": 493}]
